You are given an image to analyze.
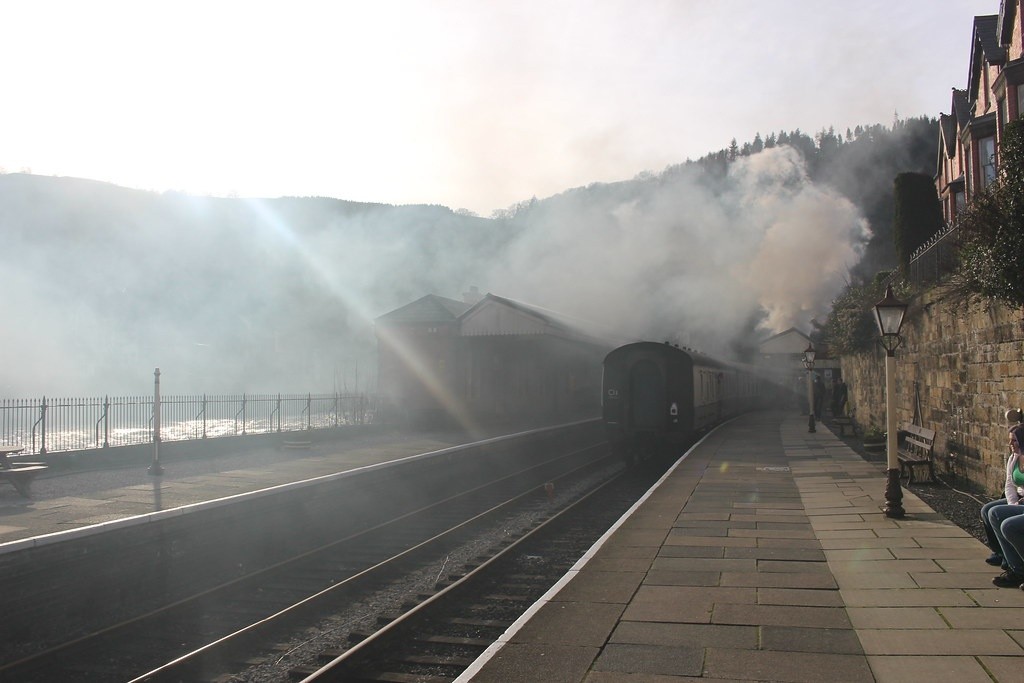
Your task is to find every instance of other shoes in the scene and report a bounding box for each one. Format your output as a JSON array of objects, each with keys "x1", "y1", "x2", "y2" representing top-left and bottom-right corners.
[
  {"x1": 992, "y1": 571, "x2": 1020, "y2": 588},
  {"x1": 1019, "y1": 583, "x2": 1024, "y2": 591},
  {"x1": 999, "y1": 557, "x2": 1010, "y2": 570},
  {"x1": 986, "y1": 552, "x2": 1003, "y2": 565}
]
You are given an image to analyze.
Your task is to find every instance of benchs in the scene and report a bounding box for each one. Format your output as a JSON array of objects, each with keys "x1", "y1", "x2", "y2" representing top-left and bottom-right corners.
[
  {"x1": 883, "y1": 422, "x2": 936, "y2": 485},
  {"x1": 0, "y1": 461, "x2": 48, "y2": 479},
  {"x1": 833, "y1": 406, "x2": 857, "y2": 437}
]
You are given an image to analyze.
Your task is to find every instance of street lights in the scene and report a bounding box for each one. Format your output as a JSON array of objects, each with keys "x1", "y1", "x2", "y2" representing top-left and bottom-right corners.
[
  {"x1": 802, "y1": 342, "x2": 818, "y2": 433},
  {"x1": 872, "y1": 282, "x2": 911, "y2": 519}
]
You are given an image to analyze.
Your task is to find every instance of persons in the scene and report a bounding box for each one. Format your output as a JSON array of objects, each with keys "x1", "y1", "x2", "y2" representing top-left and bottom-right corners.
[
  {"x1": 980, "y1": 422, "x2": 1024, "y2": 589},
  {"x1": 830, "y1": 378, "x2": 848, "y2": 417},
  {"x1": 813, "y1": 376, "x2": 823, "y2": 419},
  {"x1": 796, "y1": 377, "x2": 808, "y2": 416}
]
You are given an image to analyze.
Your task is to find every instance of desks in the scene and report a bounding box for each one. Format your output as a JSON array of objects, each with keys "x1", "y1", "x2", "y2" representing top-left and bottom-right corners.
[{"x1": 0, "y1": 446, "x2": 32, "y2": 498}]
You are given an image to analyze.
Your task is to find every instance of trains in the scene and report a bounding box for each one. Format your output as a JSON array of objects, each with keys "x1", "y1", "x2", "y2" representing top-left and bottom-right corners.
[{"x1": 600, "y1": 338, "x2": 760, "y2": 466}]
